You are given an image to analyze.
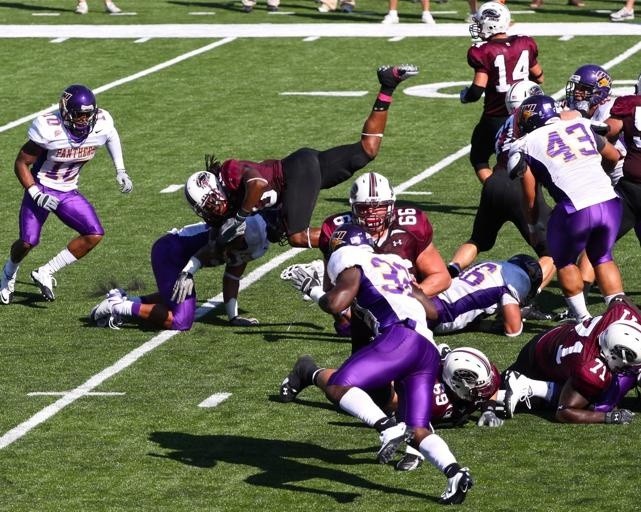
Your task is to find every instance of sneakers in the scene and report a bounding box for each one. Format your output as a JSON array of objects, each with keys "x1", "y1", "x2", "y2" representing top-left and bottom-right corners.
[
  {"x1": 279, "y1": 355, "x2": 315, "y2": 402},
  {"x1": 503, "y1": 370, "x2": 528, "y2": 419},
  {"x1": 30, "y1": 267, "x2": 58, "y2": 302},
  {"x1": 436, "y1": 467, "x2": 473, "y2": 505},
  {"x1": 0, "y1": 264, "x2": 17, "y2": 305},
  {"x1": 266, "y1": 5, "x2": 276, "y2": 11},
  {"x1": 91, "y1": 288, "x2": 127, "y2": 327},
  {"x1": 381, "y1": 9, "x2": 399, "y2": 24},
  {"x1": 240, "y1": 5, "x2": 254, "y2": 12},
  {"x1": 75, "y1": 3, "x2": 88, "y2": 15},
  {"x1": 610, "y1": 7, "x2": 635, "y2": 21},
  {"x1": 280, "y1": 258, "x2": 325, "y2": 301},
  {"x1": 422, "y1": 11, "x2": 435, "y2": 24},
  {"x1": 377, "y1": 64, "x2": 419, "y2": 88},
  {"x1": 520, "y1": 303, "x2": 578, "y2": 322},
  {"x1": 318, "y1": 3, "x2": 330, "y2": 13},
  {"x1": 105, "y1": 3, "x2": 122, "y2": 13},
  {"x1": 377, "y1": 422, "x2": 425, "y2": 472},
  {"x1": 340, "y1": 3, "x2": 353, "y2": 12}
]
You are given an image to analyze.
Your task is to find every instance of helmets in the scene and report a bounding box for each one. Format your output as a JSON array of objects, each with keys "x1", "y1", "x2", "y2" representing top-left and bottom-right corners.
[
  {"x1": 565, "y1": 64, "x2": 612, "y2": 110},
  {"x1": 505, "y1": 80, "x2": 561, "y2": 135},
  {"x1": 442, "y1": 346, "x2": 501, "y2": 406},
  {"x1": 349, "y1": 172, "x2": 396, "y2": 233},
  {"x1": 507, "y1": 253, "x2": 543, "y2": 308},
  {"x1": 469, "y1": 1, "x2": 511, "y2": 41},
  {"x1": 59, "y1": 84, "x2": 98, "y2": 143},
  {"x1": 185, "y1": 171, "x2": 233, "y2": 227},
  {"x1": 599, "y1": 320, "x2": 641, "y2": 378}
]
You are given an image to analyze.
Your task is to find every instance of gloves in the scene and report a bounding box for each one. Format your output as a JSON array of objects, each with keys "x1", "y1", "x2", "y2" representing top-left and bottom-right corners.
[
  {"x1": 170, "y1": 272, "x2": 194, "y2": 304},
  {"x1": 228, "y1": 316, "x2": 259, "y2": 326},
  {"x1": 220, "y1": 218, "x2": 246, "y2": 243},
  {"x1": 573, "y1": 101, "x2": 590, "y2": 117},
  {"x1": 33, "y1": 191, "x2": 60, "y2": 212},
  {"x1": 477, "y1": 406, "x2": 504, "y2": 427},
  {"x1": 604, "y1": 406, "x2": 635, "y2": 424},
  {"x1": 117, "y1": 173, "x2": 133, "y2": 193}
]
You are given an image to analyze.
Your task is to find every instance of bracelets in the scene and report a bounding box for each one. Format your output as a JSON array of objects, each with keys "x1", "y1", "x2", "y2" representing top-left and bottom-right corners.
[{"x1": 28, "y1": 185, "x2": 41, "y2": 199}]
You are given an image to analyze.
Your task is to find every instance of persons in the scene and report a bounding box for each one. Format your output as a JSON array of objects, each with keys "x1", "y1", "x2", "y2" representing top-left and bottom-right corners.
[
  {"x1": 92, "y1": 64, "x2": 544, "y2": 336},
  {"x1": 446, "y1": 65, "x2": 641, "y2": 423},
  {"x1": 279, "y1": 171, "x2": 507, "y2": 504},
  {"x1": 0, "y1": 86, "x2": 133, "y2": 305},
  {"x1": 76, "y1": 0, "x2": 639, "y2": 23}
]
